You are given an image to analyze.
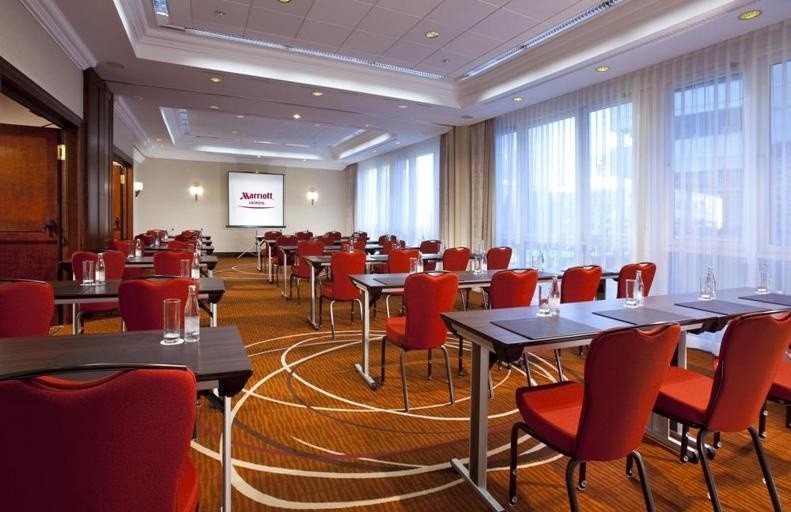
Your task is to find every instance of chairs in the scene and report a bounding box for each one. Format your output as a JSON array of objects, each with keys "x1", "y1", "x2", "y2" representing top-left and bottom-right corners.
[
  {"x1": 348, "y1": 263, "x2": 791, "y2": 511},
  {"x1": 3, "y1": 227, "x2": 252, "y2": 510},
  {"x1": 257, "y1": 228, "x2": 514, "y2": 336}
]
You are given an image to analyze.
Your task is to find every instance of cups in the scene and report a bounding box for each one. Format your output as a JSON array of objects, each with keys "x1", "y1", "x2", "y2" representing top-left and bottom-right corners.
[
  {"x1": 473, "y1": 255, "x2": 481, "y2": 274},
  {"x1": 625, "y1": 279, "x2": 638, "y2": 308},
  {"x1": 699, "y1": 273, "x2": 711, "y2": 300},
  {"x1": 179, "y1": 259, "x2": 189, "y2": 279},
  {"x1": 755, "y1": 270, "x2": 768, "y2": 294},
  {"x1": 537, "y1": 285, "x2": 549, "y2": 314},
  {"x1": 81, "y1": 260, "x2": 94, "y2": 284},
  {"x1": 162, "y1": 298, "x2": 180, "y2": 344},
  {"x1": 127, "y1": 242, "x2": 134, "y2": 258},
  {"x1": 409, "y1": 257, "x2": 417, "y2": 275}
]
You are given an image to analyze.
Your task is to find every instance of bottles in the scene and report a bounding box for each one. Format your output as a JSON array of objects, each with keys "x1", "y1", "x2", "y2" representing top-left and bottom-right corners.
[
  {"x1": 416, "y1": 252, "x2": 423, "y2": 274},
  {"x1": 631, "y1": 270, "x2": 645, "y2": 307},
  {"x1": 706, "y1": 266, "x2": 717, "y2": 300},
  {"x1": 190, "y1": 252, "x2": 200, "y2": 282},
  {"x1": 477, "y1": 244, "x2": 487, "y2": 275},
  {"x1": 547, "y1": 274, "x2": 560, "y2": 315},
  {"x1": 182, "y1": 285, "x2": 200, "y2": 343},
  {"x1": 94, "y1": 253, "x2": 105, "y2": 286},
  {"x1": 134, "y1": 238, "x2": 142, "y2": 258}
]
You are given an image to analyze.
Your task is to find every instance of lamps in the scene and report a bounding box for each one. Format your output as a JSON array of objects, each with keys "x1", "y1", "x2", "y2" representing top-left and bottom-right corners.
[
  {"x1": 134, "y1": 181, "x2": 143, "y2": 198},
  {"x1": 194, "y1": 182, "x2": 198, "y2": 202},
  {"x1": 309, "y1": 188, "x2": 316, "y2": 205}
]
